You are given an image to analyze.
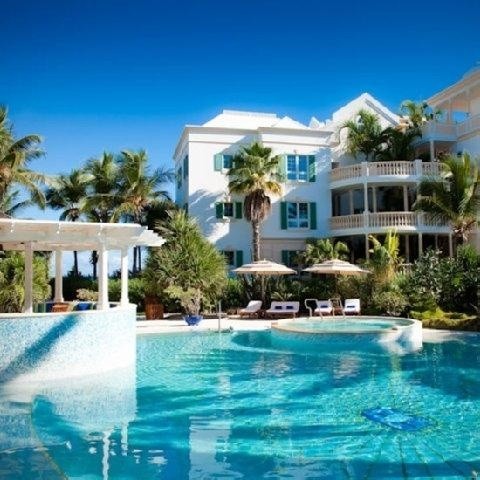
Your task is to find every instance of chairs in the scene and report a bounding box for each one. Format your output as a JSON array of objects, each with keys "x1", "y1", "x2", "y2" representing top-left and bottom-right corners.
[{"x1": 238, "y1": 298, "x2": 362, "y2": 319}]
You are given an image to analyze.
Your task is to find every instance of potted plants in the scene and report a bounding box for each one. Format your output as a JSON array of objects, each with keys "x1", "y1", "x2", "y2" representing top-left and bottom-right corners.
[
  {"x1": 141, "y1": 268, "x2": 164, "y2": 320},
  {"x1": 164, "y1": 282, "x2": 204, "y2": 326}
]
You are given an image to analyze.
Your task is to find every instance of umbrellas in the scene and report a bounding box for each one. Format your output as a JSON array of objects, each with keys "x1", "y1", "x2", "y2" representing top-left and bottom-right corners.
[
  {"x1": 230, "y1": 259, "x2": 298, "y2": 309},
  {"x1": 302, "y1": 258, "x2": 373, "y2": 306}
]
